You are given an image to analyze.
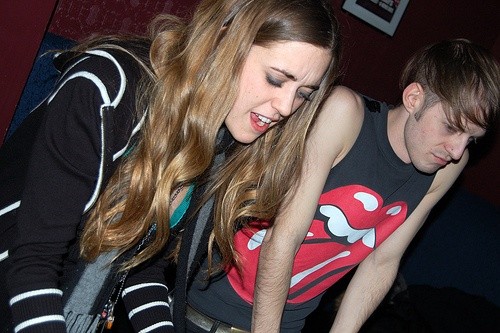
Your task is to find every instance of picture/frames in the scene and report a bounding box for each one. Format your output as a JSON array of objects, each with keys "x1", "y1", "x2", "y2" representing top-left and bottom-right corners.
[{"x1": 339, "y1": 0, "x2": 410, "y2": 39}]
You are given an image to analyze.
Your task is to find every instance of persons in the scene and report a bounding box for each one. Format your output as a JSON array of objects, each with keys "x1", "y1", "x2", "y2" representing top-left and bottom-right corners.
[
  {"x1": 0, "y1": 0, "x2": 342, "y2": 333},
  {"x1": 182, "y1": 36, "x2": 499, "y2": 332}
]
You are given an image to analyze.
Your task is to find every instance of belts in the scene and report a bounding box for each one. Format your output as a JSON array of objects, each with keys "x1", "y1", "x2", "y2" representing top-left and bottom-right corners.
[{"x1": 185, "y1": 304, "x2": 251, "y2": 333}]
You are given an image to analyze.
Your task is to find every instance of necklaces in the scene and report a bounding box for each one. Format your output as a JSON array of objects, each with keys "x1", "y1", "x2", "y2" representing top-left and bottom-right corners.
[{"x1": 100, "y1": 180, "x2": 187, "y2": 330}]
